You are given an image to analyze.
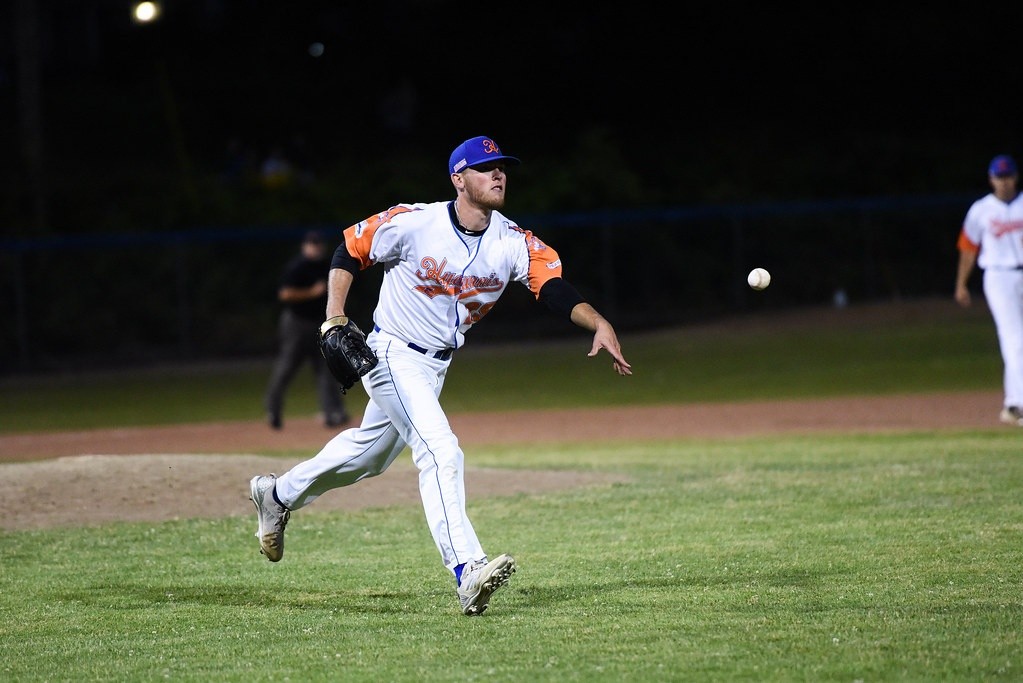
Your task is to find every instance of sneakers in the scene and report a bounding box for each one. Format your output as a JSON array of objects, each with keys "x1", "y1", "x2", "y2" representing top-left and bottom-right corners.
[
  {"x1": 456, "y1": 553, "x2": 516, "y2": 617},
  {"x1": 248, "y1": 473, "x2": 291, "y2": 562}
]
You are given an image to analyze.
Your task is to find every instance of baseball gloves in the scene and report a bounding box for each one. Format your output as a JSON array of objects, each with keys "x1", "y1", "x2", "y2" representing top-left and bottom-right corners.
[{"x1": 316, "y1": 315, "x2": 379, "y2": 391}]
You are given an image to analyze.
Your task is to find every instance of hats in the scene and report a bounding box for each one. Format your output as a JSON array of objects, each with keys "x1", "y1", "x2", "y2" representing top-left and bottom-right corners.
[
  {"x1": 448, "y1": 136, "x2": 522, "y2": 175},
  {"x1": 303, "y1": 227, "x2": 326, "y2": 246},
  {"x1": 988, "y1": 155, "x2": 1013, "y2": 178}
]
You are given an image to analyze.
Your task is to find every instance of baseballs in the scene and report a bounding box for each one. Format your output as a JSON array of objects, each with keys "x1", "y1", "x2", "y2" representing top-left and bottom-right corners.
[{"x1": 748, "y1": 268, "x2": 771, "y2": 291}]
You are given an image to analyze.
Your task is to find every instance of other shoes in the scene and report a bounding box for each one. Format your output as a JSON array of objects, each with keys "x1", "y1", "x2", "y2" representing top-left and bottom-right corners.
[
  {"x1": 324, "y1": 408, "x2": 348, "y2": 427},
  {"x1": 998, "y1": 406, "x2": 1023, "y2": 426},
  {"x1": 268, "y1": 408, "x2": 283, "y2": 431}
]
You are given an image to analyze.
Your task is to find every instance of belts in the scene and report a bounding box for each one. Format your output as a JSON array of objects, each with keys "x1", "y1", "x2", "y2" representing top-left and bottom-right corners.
[{"x1": 408, "y1": 342, "x2": 455, "y2": 361}]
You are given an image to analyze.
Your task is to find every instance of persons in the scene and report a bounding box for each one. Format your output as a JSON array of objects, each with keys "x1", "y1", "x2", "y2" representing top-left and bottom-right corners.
[
  {"x1": 264, "y1": 231, "x2": 351, "y2": 429},
  {"x1": 249, "y1": 136, "x2": 633, "y2": 616},
  {"x1": 956, "y1": 156, "x2": 1023, "y2": 428}
]
